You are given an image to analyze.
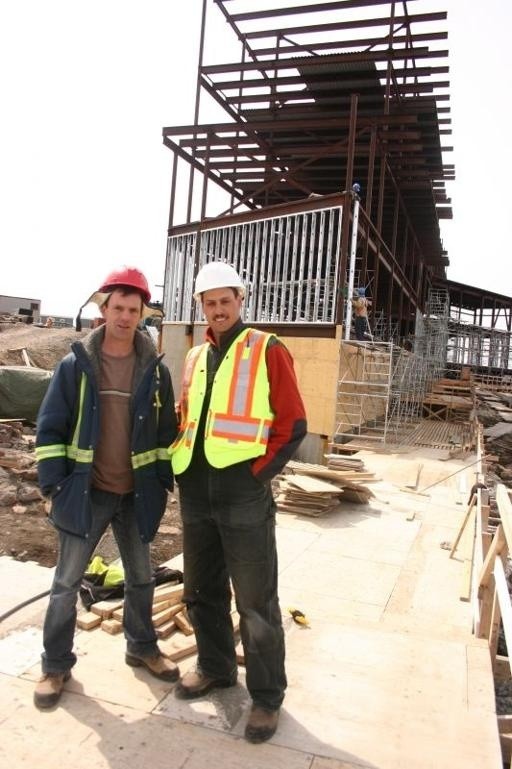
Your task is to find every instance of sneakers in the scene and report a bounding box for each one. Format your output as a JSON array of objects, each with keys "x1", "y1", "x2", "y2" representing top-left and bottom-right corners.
[
  {"x1": 179, "y1": 667, "x2": 237, "y2": 697},
  {"x1": 246, "y1": 701, "x2": 280, "y2": 740},
  {"x1": 125, "y1": 649, "x2": 181, "y2": 681},
  {"x1": 33, "y1": 668, "x2": 72, "y2": 708}
]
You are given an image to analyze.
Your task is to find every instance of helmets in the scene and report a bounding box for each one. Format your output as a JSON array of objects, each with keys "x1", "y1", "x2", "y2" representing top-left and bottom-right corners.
[
  {"x1": 194, "y1": 262, "x2": 246, "y2": 301},
  {"x1": 96, "y1": 267, "x2": 152, "y2": 304}
]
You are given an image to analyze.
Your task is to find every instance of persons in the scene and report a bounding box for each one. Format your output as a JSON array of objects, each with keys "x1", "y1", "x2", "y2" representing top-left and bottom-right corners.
[
  {"x1": 33, "y1": 266, "x2": 179, "y2": 708},
  {"x1": 353, "y1": 184, "x2": 361, "y2": 192},
  {"x1": 163, "y1": 262, "x2": 307, "y2": 744},
  {"x1": 350, "y1": 288, "x2": 369, "y2": 340}
]
